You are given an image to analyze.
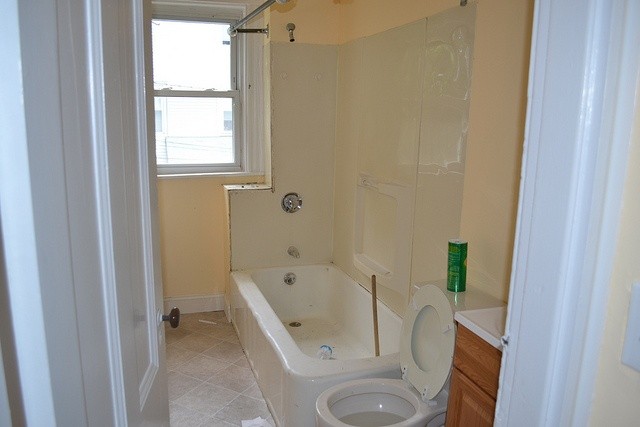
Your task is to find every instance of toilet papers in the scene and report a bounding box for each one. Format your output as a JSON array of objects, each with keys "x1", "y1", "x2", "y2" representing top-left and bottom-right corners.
[{"x1": 444, "y1": 236, "x2": 470, "y2": 293}]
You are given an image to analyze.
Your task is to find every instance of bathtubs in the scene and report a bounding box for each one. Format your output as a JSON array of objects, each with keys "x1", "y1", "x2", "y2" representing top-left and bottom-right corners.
[{"x1": 227, "y1": 261, "x2": 404, "y2": 426}]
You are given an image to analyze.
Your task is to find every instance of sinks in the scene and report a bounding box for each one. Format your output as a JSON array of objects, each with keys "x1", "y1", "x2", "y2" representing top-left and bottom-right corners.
[{"x1": 454, "y1": 306, "x2": 506, "y2": 354}]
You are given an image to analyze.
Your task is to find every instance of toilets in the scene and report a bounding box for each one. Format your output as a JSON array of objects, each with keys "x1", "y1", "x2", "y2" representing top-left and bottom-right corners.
[{"x1": 311, "y1": 284, "x2": 456, "y2": 427}]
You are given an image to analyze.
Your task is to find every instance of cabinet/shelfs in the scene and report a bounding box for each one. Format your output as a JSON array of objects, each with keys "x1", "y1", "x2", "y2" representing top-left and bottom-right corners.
[{"x1": 444, "y1": 321, "x2": 503, "y2": 426}]
